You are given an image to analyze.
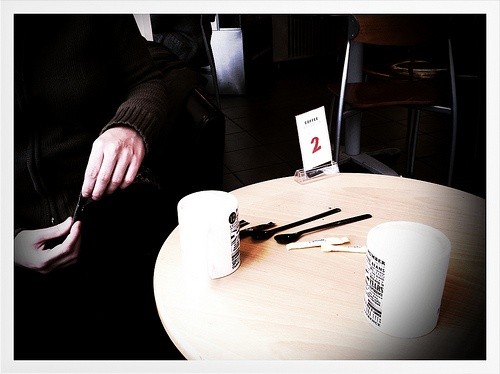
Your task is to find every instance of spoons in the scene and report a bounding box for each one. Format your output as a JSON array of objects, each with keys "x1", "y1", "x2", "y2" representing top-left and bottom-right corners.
[
  {"x1": 274, "y1": 213, "x2": 372, "y2": 244},
  {"x1": 251, "y1": 208, "x2": 341, "y2": 241}
]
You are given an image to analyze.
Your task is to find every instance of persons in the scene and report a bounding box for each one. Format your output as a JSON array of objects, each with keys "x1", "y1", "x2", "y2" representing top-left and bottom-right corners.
[{"x1": 15, "y1": 13, "x2": 210, "y2": 361}]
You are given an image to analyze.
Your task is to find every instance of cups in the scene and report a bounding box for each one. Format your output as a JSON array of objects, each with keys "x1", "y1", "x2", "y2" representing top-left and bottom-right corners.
[
  {"x1": 176, "y1": 190, "x2": 242, "y2": 279},
  {"x1": 363, "y1": 221, "x2": 452, "y2": 339}
]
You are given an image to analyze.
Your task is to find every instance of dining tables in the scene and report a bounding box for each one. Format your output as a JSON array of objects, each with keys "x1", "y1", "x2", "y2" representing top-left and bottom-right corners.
[{"x1": 150, "y1": 171, "x2": 486, "y2": 361}]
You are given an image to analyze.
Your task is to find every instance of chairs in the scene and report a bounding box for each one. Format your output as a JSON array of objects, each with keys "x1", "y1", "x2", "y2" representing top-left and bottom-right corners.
[
  {"x1": 326, "y1": 15, "x2": 436, "y2": 179},
  {"x1": 409, "y1": 15, "x2": 485, "y2": 187}
]
